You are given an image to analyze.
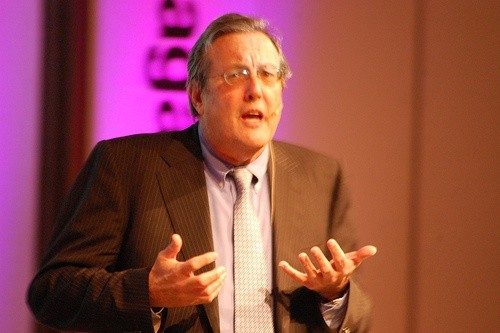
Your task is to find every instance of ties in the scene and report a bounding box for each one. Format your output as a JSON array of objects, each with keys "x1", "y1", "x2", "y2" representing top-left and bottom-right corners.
[{"x1": 228, "y1": 168, "x2": 274, "y2": 333}]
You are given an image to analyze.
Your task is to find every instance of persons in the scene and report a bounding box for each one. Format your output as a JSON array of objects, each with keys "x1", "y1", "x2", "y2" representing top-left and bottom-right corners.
[{"x1": 26, "y1": 14, "x2": 377, "y2": 333}]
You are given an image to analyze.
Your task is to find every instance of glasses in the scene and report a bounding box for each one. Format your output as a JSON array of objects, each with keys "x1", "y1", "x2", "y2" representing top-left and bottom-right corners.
[{"x1": 198, "y1": 66, "x2": 285, "y2": 87}]
